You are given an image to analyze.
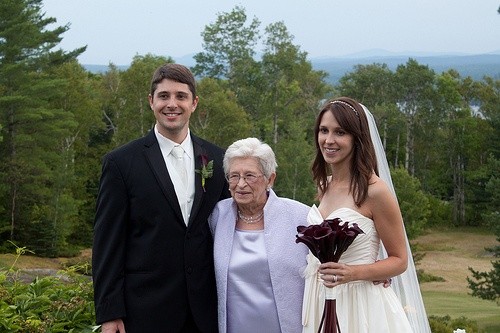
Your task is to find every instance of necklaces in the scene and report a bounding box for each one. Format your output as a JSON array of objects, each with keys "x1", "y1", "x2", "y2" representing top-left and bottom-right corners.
[{"x1": 237, "y1": 209, "x2": 264, "y2": 224}]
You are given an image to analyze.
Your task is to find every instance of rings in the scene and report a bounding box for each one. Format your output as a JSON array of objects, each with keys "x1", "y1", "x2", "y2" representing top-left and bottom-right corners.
[{"x1": 333, "y1": 274, "x2": 337, "y2": 282}]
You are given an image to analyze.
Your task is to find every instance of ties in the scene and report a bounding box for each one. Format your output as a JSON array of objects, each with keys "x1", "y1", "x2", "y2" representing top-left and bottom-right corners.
[{"x1": 170, "y1": 146, "x2": 188, "y2": 227}]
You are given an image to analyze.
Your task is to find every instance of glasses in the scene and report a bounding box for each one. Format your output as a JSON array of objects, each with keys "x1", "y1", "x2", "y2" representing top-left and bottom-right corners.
[{"x1": 225, "y1": 172, "x2": 264, "y2": 183}]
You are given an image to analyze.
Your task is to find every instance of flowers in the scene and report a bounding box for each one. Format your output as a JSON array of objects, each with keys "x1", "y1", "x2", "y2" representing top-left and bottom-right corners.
[
  {"x1": 194, "y1": 153, "x2": 214, "y2": 192},
  {"x1": 295, "y1": 217, "x2": 366, "y2": 333}
]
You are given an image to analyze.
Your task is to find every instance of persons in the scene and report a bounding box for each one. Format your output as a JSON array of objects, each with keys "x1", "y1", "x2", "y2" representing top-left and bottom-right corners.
[
  {"x1": 300, "y1": 96, "x2": 432, "y2": 333},
  {"x1": 208, "y1": 137, "x2": 316, "y2": 333},
  {"x1": 92, "y1": 64, "x2": 233, "y2": 333}
]
